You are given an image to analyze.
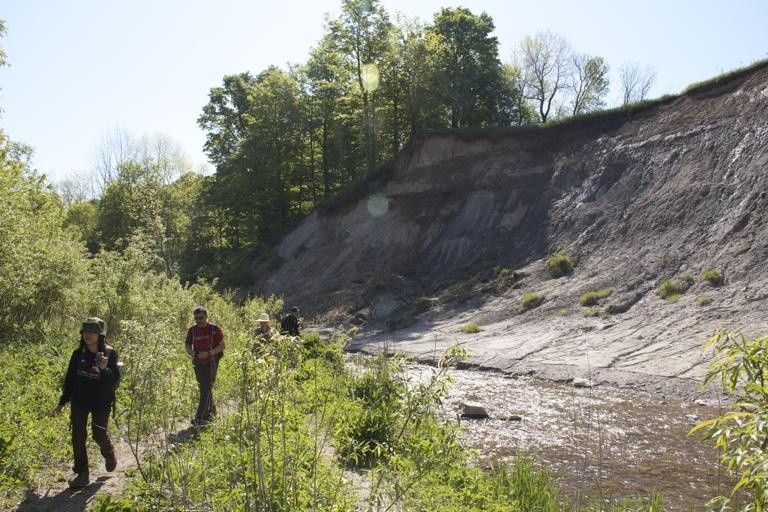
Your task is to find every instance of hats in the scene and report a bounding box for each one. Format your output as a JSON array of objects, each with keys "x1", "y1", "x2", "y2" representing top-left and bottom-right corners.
[
  {"x1": 78, "y1": 317, "x2": 108, "y2": 339},
  {"x1": 255, "y1": 313, "x2": 269, "y2": 323}
]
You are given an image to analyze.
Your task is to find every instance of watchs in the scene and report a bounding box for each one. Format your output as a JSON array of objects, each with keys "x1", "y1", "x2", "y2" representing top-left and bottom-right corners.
[{"x1": 207, "y1": 350, "x2": 212, "y2": 358}]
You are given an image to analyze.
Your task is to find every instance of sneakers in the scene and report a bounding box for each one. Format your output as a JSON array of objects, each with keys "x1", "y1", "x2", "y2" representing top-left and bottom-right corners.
[
  {"x1": 67, "y1": 473, "x2": 89, "y2": 491},
  {"x1": 190, "y1": 419, "x2": 207, "y2": 427},
  {"x1": 106, "y1": 453, "x2": 116, "y2": 472}
]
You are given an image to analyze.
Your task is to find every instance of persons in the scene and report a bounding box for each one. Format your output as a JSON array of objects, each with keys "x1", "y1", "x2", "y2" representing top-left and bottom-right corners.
[
  {"x1": 280, "y1": 306, "x2": 302, "y2": 337},
  {"x1": 184, "y1": 306, "x2": 226, "y2": 426},
  {"x1": 50, "y1": 315, "x2": 120, "y2": 490},
  {"x1": 251, "y1": 312, "x2": 277, "y2": 356}
]
